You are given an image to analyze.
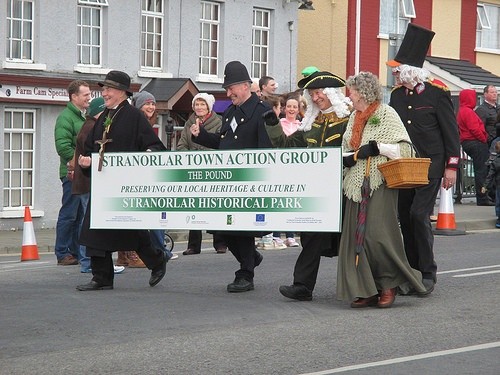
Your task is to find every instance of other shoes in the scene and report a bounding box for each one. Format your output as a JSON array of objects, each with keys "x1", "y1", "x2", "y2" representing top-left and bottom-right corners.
[
  {"x1": 273, "y1": 238, "x2": 284, "y2": 244},
  {"x1": 217, "y1": 248, "x2": 226, "y2": 253},
  {"x1": 477, "y1": 196, "x2": 496, "y2": 205},
  {"x1": 113, "y1": 265, "x2": 124, "y2": 273},
  {"x1": 496, "y1": 218, "x2": 500, "y2": 227},
  {"x1": 257, "y1": 242, "x2": 287, "y2": 250},
  {"x1": 227, "y1": 251, "x2": 263, "y2": 292},
  {"x1": 170, "y1": 253, "x2": 178, "y2": 260},
  {"x1": 58, "y1": 255, "x2": 79, "y2": 265},
  {"x1": 351, "y1": 287, "x2": 397, "y2": 308},
  {"x1": 183, "y1": 248, "x2": 197, "y2": 255},
  {"x1": 419, "y1": 279, "x2": 433, "y2": 295}
]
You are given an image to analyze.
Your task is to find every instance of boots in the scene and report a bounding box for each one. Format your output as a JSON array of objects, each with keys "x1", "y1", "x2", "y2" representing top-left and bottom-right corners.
[{"x1": 117, "y1": 251, "x2": 146, "y2": 268}]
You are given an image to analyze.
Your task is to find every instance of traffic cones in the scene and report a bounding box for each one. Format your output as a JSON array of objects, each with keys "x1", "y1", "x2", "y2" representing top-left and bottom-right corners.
[
  {"x1": 431, "y1": 177, "x2": 468, "y2": 236},
  {"x1": 20, "y1": 206, "x2": 41, "y2": 261}
]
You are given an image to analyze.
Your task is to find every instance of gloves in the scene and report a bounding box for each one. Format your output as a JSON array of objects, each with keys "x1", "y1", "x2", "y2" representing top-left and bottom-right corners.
[
  {"x1": 263, "y1": 110, "x2": 277, "y2": 125},
  {"x1": 343, "y1": 140, "x2": 379, "y2": 168}
]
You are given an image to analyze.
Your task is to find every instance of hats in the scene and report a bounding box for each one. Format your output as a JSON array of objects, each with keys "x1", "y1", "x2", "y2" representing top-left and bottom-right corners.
[
  {"x1": 386, "y1": 23, "x2": 436, "y2": 87},
  {"x1": 222, "y1": 61, "x2": 253, "y2": 88},
  {"x1": 131, "y1": 91, "x2": 156, "y2": 110},
  {"x1": 298, "y1": 67, "x2": 352, "y2": 132},
  {"x1": 98, "y1": 70, "x2": 133, "y2": 96},
  {"x1": 192, "y1": 93, "x2": 215, "y2": 113},
  {"x1": 89, "y1": 97, "x2": 105, "y2": 116}
]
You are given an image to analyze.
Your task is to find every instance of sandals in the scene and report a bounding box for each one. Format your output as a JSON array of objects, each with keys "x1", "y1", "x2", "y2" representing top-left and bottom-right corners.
[{"x1": 286, "y1": 238, "x2": 299, "y2": 247}]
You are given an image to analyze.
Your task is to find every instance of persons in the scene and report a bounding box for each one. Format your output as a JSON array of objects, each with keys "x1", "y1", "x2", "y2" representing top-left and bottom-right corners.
[{"x1": 51, "y1": 21, "x2": 500, "y2": 307}]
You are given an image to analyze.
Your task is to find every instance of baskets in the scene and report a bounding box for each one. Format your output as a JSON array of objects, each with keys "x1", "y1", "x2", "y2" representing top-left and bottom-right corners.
[{"x1": 377, "y1": 139, "x2": 432, "y2": 187}]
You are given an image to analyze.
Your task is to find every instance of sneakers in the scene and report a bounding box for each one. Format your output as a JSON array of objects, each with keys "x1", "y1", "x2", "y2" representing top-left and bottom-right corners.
[{"x1": 279, "y1": 283, "x2": 312, "y2": 301}]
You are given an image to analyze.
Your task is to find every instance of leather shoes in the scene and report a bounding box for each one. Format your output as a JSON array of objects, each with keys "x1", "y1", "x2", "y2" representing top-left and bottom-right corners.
[
  {"x1": 77, "y1": 280, "x2": 114, "y2": 291},
  {"x1": 149, "y1": 251, "x2": 166, "y2": 287}
]
